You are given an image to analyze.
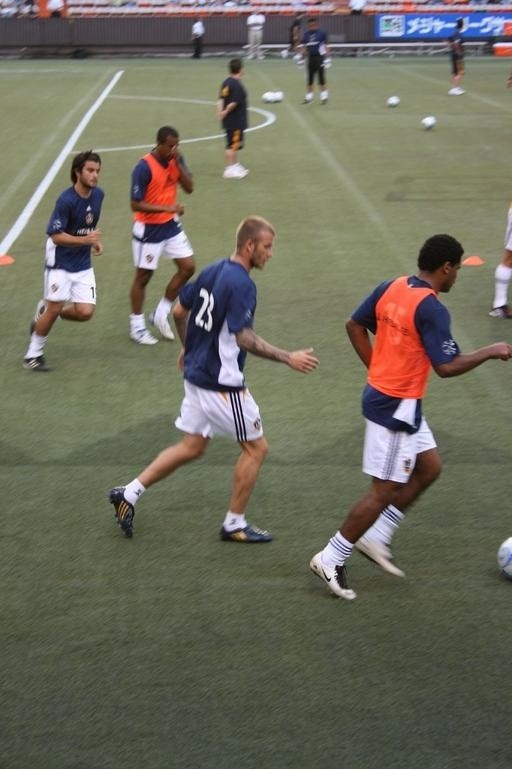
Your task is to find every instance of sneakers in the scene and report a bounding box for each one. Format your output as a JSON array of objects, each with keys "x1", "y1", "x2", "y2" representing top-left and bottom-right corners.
[
  {"x1": 26, "y1": 297, "x2": 47, "y2": 336},
  {"x1": 297, "y1": 97, "x2": 313, "y2": 106},
  {"x1": 127, "y1": 328, "x2": 160, "y2": 347},
  {"x1": 291, "y1": 53, "x2": 303, "y2": 63},
  {"x1": 247, "y1": 55, "x2": 255, "y2": 61},
  {"x1": 257, "y1": 55, "x2": 266, "y2": 61},
  {"x1": 279, "y1": 49, "x2": 288, "y2": 60},
  {"x1": 218, "y1": 520, "x2": 277, "y2": 547},
  {"x1": 306, "y1": 550, "x2": 358, "y2": 603},
  {"x1": 356, "y1": 538, "x2": 407, "y2": 582},
  {"x1": 446, "y1": 85, "x2": 468, "y2": 98},
  {"x1": 319, "y1": 99, "x2": 329, "y2": 106},
  {"x1": 20, "y1": 353, "x2": 58, "y2": 373},
  {"x1": 146, "y1": 312, "x2": 176, "y2": 343},
  {"x1": 220, "y1": 167, "x2": 245, "y2": 181},
  {"x1": 235, "y1": 163, "x2": 249, "y2": 175},
  {"x1": 106, "y1": 482, "x2": 139, "y2": 543}
]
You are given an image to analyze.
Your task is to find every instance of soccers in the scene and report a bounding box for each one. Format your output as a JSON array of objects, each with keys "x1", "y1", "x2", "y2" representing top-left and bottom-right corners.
[
  {"x1": 261, "y1": 91, "x2": 284, "y2": 103},
  {"x1": 386, "y1": 95, "x2": 402, "y2": 109},
  {"x1": 420, "y1": 115, "x2": 438, "y2": 132},
  {"x1": 496, "y1": 535, "x2": 512, "y2": 582}
]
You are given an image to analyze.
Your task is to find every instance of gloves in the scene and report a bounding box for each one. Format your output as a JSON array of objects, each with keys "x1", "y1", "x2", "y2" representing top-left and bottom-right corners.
[{"x1": 321, "y1": 58, "x2": 332, "y2": 70}]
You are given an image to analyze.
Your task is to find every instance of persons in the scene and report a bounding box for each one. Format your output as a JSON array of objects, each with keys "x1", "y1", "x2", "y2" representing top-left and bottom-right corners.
[
  {"x1": 108, "y1": 215, "x2": 318, "y2": 543},
  {"x1": 295, "y1": 18, "x2": 331, "y2": 103},
  {"x1": 310, "y1": 234, "x2": 512, "y2": 599},
  {"x1": 217, "y1": 59, "x2": 249, "y2": 179},
  {"x1": 246, "y1": 8, "x2": 266, "y2": 62},
  {"x1": 450, "y1": 19, "x2": 467, "y2": 95},
  {"x1": 492, "y1": 201, "x2": 512, "y2": 318},
  {"x1": 130, "y1": 126, "x2": 194, "y2": 346},
  {"x1": 191, "y1": 17, "x2": 205, "y2": 59},
  {"x1": 281, "y1": 11, "x2": 306, "y2": 58},
  {"x1": 22, "y1": 152, "x2": 104, "y2": 371}
]
